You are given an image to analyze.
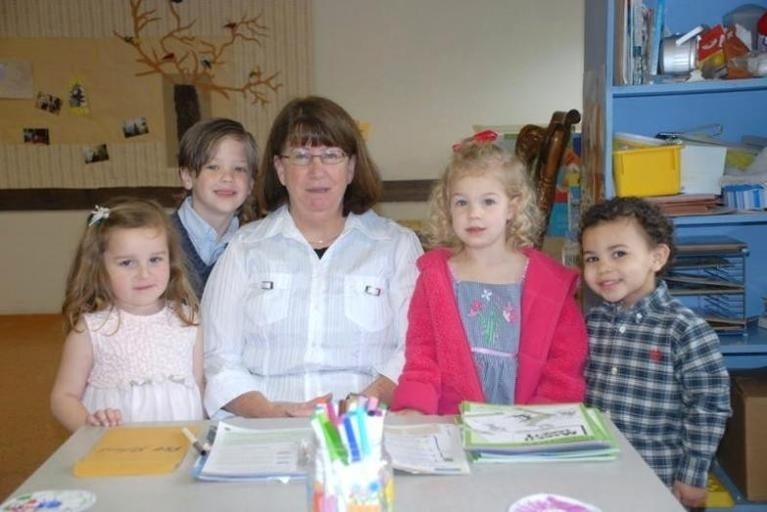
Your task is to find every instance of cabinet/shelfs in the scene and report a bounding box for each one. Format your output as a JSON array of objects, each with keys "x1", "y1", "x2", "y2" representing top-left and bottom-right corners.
[{"x1": 583, "y1": 2, "x2": 767, "y2": 512}]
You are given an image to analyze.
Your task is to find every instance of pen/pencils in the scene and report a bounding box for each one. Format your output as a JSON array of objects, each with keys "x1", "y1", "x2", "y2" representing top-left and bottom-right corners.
[
  {"x1": 309, "y1": 394, "x2": 388, "y2": 512},
  {"x1": 182, "y1": 426, "x2": 207, "y2": 455}
]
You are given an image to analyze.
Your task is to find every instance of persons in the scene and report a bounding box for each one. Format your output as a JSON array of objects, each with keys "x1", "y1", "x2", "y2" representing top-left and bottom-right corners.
[
  {"x1": 189, "y1": 94, "x2": 427, "y2": 420},
  {"x1": 161, "y1": 118, "x2": 263, "y2": 304},
  {"x1": 382, "y1": 129, "x2": 590, "y2": 414},
  {"x1": 567, "y1": 193, "x2": 736, "y2": 512},
  {"x1": 47, "y1": 193, "x2": 209, "y2": 436}
]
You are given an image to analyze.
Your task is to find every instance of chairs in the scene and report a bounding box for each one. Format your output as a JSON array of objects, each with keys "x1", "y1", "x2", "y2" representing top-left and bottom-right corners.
[{"x1": 514, "y1": 110, "x2": 580, "y2": 252}]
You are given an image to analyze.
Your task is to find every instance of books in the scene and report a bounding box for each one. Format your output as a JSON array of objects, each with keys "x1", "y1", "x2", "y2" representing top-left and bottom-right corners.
[
  {"x1": 645, "y1": 192, "x2": 748, "y2": 334},
  {"x1": 612, "y1": 0, "x2": 665, "y2": 86},
  {"x1": 72, "y1": 400, "x2": 621, "y2": 483}
]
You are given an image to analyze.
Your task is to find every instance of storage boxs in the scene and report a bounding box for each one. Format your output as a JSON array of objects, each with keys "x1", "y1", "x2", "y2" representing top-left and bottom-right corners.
[
  {"x1": 715, "y1": 366, "x2": 767, "y2": 502},
  {"x1": 613, "y1": 144, "x2": 681, "y2": 199}
]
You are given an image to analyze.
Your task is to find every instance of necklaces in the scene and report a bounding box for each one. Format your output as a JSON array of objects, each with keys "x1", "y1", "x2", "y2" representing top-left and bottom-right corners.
[{"x1": 305, "y1": 234, "x2": 341, "y2": 247}]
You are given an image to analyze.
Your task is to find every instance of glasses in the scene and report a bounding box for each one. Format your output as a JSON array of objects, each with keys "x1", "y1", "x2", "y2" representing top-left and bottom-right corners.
[{"x1": 279, "y1": 147, "x2": 354, "y2": 165}]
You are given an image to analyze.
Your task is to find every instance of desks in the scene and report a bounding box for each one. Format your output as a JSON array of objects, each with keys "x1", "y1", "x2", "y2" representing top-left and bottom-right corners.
[{"x1": 0, "y1": 407, "x2": 683, "y2": 511}]
